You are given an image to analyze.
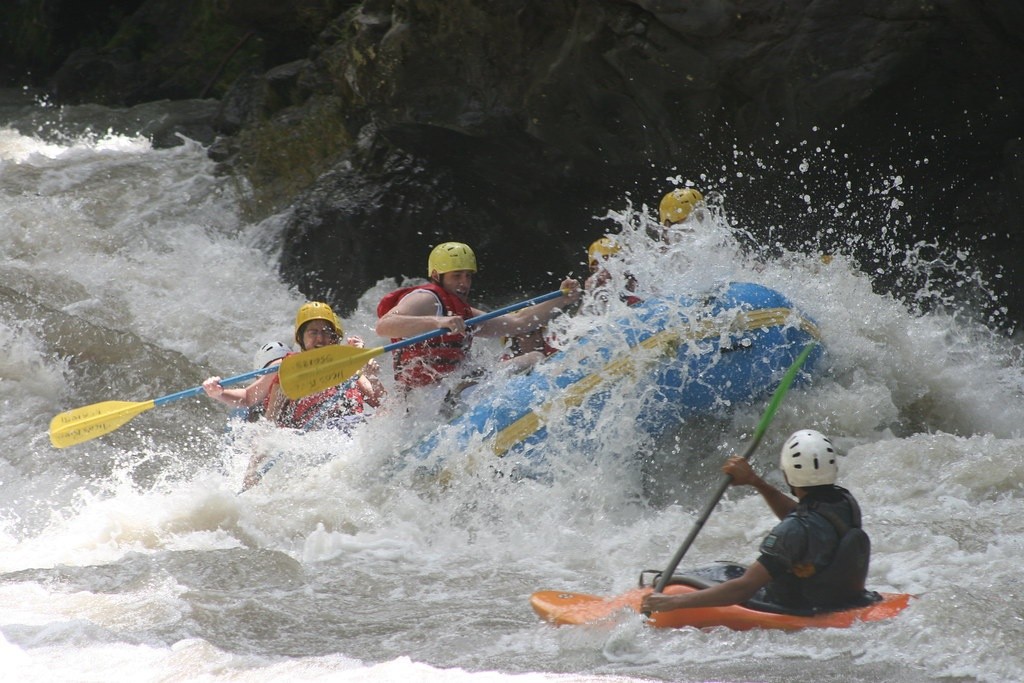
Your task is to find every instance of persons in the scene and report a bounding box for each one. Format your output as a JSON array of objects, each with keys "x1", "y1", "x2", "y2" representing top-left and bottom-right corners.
[
  {"x1": 201, "y1": 302, "x2": 386, "y2": 491},
  {"x1": 376, "y1": 187, "x2": 705, "y2": 387},
  {"x1": 639, "y1": 428, "x2": 871, "y2": 618}
]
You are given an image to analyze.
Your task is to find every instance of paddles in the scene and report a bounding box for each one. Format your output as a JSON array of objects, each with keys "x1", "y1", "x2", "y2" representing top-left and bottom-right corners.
[
  {"x1": 275, "y1": 289, "x2": 570, "y2": 400},
  {"x1": 635, "y1": 343, "x2": 817, "y2": 633},
  {"x1": 47, "y1": 365, "x2": 282, "y2": 448}
]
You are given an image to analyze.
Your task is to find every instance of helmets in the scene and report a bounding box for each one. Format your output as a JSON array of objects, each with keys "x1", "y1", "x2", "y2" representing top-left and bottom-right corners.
[
  {"x1": 332, "y1": 311, "x2": 343, "y2": 336},
  {"x1": 427, "y1": 242, "x2": 477, "y2": 279},
  {"x1": 295, "y1": 301, "x2": 336, "y2": 344},
  {"x1": 254, "y1": 340, "x2": 289, "y2": 372},
  {"x1": 658, "y1": 187, "x2": 704, "y2": 224},
  {"x1": 589, "y1": 237, "x2": 622, "y2": 272},
  {"x1": 780, "y1": 430, "x2": 837, "y2": 487}
]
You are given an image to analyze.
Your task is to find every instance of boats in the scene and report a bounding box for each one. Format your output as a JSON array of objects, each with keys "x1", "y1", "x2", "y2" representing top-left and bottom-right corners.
[
  {"x1": 363, "y1": 276, "x2": 828, "y2": 492},
  {"x1": 531, "y1": 571, "x2": 920, "y2": 634}
]
